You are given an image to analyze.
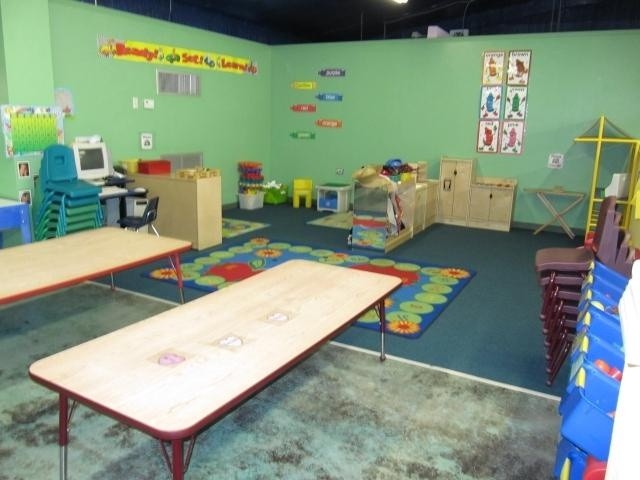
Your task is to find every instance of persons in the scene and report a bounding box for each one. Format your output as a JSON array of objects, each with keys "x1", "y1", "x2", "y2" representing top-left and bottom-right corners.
[
  {"x1": 20, "y1": 164, "x2": 28, "y2": 175},
  {"x1": 21, "y1": 193, "x2": 28, "y2": 202}
]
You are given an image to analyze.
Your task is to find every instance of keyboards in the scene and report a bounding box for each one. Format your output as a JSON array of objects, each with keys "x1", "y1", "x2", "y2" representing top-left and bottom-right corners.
[{"x1": 99, "y1": 185, "x2": 128, "y2": 197}]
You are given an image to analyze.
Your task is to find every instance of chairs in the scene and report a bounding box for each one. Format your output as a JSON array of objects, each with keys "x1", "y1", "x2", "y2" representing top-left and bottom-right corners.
[
  {"x1": 30, "y1": 144, "x2": 105, "y2": 243},
  {"x1": 116, "y1": 196, "x2": 182, "y2": 278},
  {"x1": 534, "y1": 195, "x2": 636, "y2": 388},
  {"x1": 293, "y1": 176, "x2": 314, "y2": 209}
]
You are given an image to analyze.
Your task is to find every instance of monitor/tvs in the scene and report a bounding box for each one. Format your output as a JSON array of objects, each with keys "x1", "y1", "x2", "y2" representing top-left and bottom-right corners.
[{"x1": 68, "y1": 142, "x2": 109, "y2": 186}]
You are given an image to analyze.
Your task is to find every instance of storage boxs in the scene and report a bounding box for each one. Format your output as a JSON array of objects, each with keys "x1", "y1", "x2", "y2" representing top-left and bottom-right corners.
[
  {"x1": 117, "y1": 159, "x2": 139, "y2": 173},
  {"x1": 554, "y1": 261, "x2": 631, "y2": 479},
  {"x1": 139, "y1": 161, "x2": 171, "y2": 175},
  {"x1": 237, "y1": 190, "x2": 267, "y2": 210},
  {"x1": 263, "y1": 183, "x2": 289, "y2": 206}
]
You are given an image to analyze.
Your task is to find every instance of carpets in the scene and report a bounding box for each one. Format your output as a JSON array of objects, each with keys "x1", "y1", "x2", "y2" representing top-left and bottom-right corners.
[
  {"x1": 304, "y1": 211, "x2": 355, "y2": 231},
  {"x1": 223, "y1": 216, "x2": 272, "y2": 239},
  {"x1": 139, "y1": 234, "x2": 479, "y2": 341}
]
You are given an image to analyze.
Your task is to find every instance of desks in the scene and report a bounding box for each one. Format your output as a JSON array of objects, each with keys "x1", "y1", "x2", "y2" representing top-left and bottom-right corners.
[
  {"x1": 315, "y1": 183, "x2": 353, "y2": 213},
  {"x1": 0, "y1": 225, "x2": 195, "y2": 316},
  {"x1": 1, "y1": 194, "x2": 34, "y2": 249},
  {"x1": 26, "y1": 257, "x2": 404, "y2": 479},
  {"x1": 87, "y1": 173, "x2": 136, "y2": 230},
  {"x1": 523, "y1": 184, "x2": 584, "y2": 242}
]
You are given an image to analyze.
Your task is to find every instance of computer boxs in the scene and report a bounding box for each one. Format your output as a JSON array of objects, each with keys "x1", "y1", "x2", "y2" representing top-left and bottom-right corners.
[{"x1": 126, "y1": 196, "x2": 149, "y2": 233}]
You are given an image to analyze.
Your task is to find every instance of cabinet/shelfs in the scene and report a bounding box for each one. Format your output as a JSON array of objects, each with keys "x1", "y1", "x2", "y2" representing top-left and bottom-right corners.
[
  {"x1": 414, "y1": 186, "x2": 426, "y2": 233},
  {"x1": 469, "y1": 182, "x2": 516, "y2": 232},
  {"x1": 438, "y1": 158, "x2": 474, "y2": 228},
  {"x1": 124, "y1": 173, "x2": 223, "y2": 253},
  {"x1": 350, "y1": 177, "x2": 414, "y2": 253},
  {"x1": 426, "y1": 179, "x2": 437, "y2": 229}
]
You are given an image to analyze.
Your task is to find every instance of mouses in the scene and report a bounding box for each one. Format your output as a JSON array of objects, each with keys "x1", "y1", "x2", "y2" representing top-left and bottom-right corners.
[{"x1": 134, "y1": 187, "x2": 146, "y2": 192}]
